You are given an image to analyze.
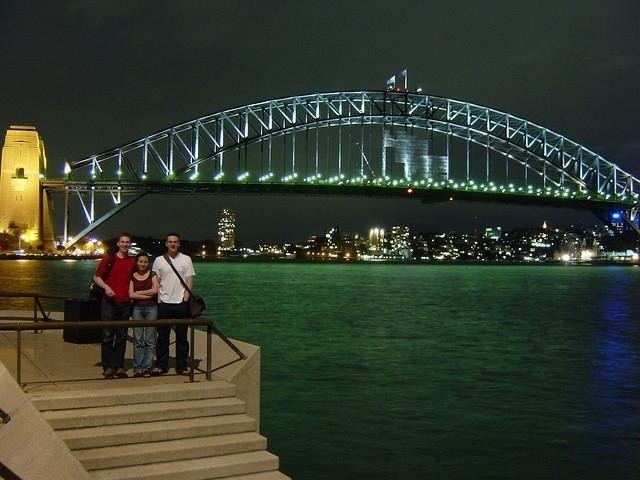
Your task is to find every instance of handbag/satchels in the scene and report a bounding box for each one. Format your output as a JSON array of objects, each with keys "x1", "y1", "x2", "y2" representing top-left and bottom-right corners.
[
  {"x1": 189, "y1": 295, "x2": 206, "y2": 318},
  {"x1": 88, "y1": 283, "x2": 105, "y2": 301}
]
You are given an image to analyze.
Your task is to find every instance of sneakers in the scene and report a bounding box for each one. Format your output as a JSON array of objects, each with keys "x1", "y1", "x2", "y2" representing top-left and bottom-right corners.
[
  {"x1": 177, "y1": 367, "x2": 190, "y2": 375},
  {"x1": 104, "y1": 368, "x2": 163, "y2": 378}
]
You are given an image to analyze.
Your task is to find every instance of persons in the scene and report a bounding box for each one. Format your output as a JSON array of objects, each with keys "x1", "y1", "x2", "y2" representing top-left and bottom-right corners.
[
  {"x1": 150, "y1": 232, "x2": 197, "y2": 377},
  {"x1": 91, "y1": 233, "x2": 139, "y2": 381},
  {"x1": 129, "y1": 251, "x2": 159, "y2": 379}
]
